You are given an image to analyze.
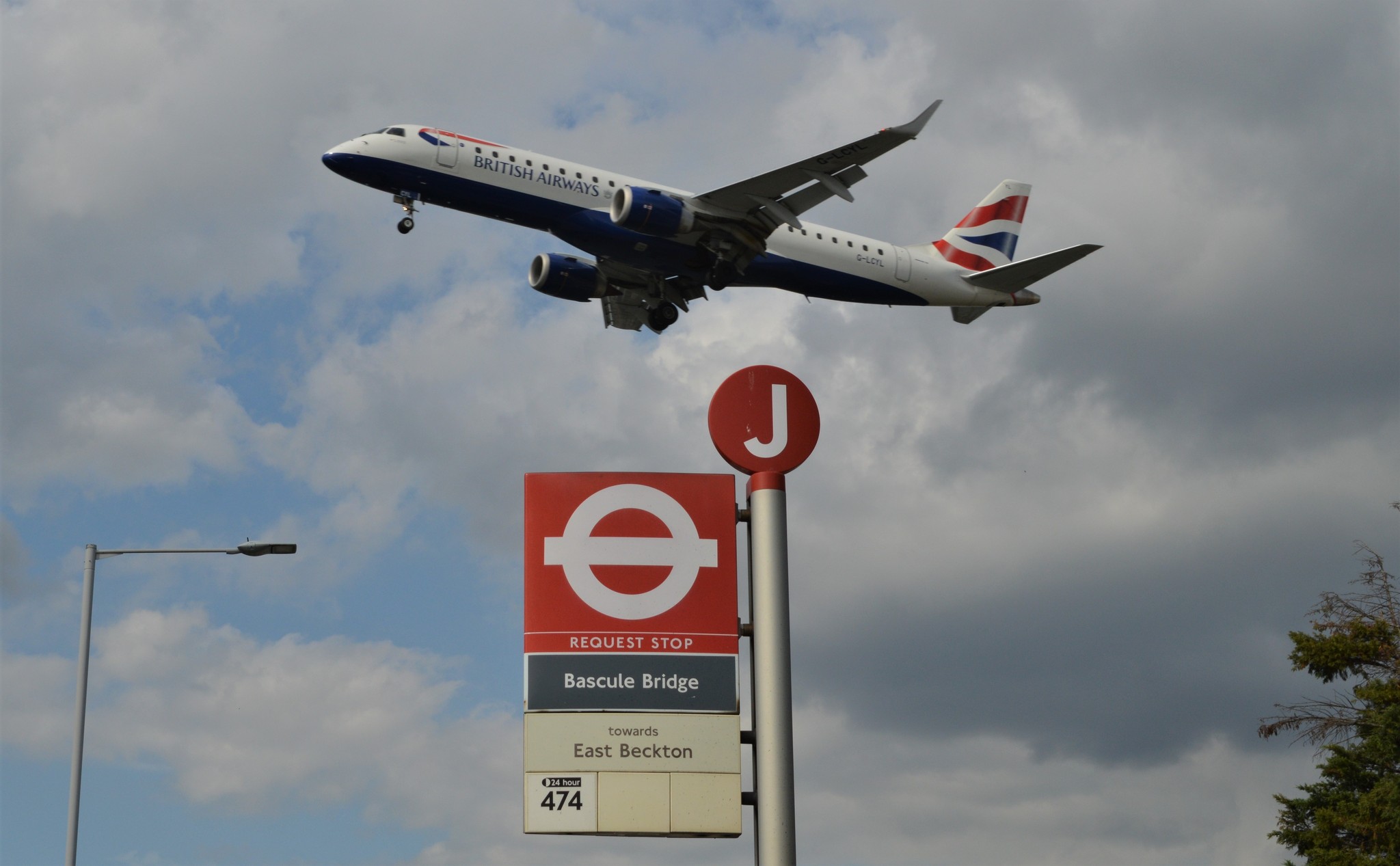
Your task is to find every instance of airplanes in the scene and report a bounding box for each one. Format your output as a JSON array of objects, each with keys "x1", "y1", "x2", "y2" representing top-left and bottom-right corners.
[{"x1": 320, "y1": 97, "x2": 1104, "y2": 335}]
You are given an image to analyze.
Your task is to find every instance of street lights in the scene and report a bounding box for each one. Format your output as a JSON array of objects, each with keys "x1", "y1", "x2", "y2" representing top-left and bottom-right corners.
[{"x1": 63, "y1": 536, "x2": 297, "y2": 866}]
